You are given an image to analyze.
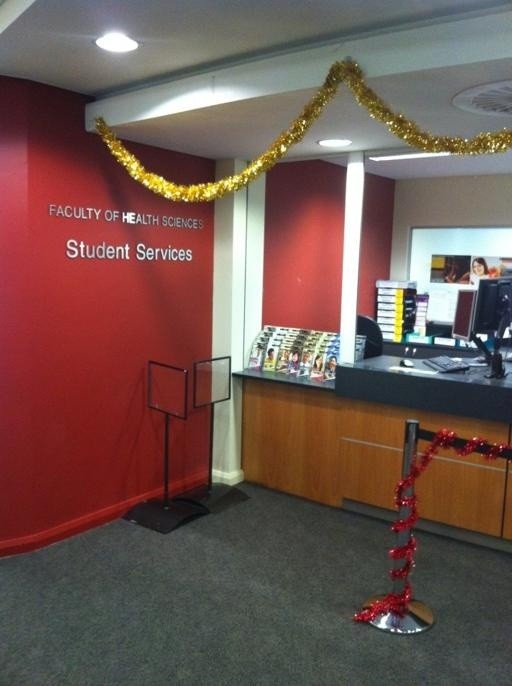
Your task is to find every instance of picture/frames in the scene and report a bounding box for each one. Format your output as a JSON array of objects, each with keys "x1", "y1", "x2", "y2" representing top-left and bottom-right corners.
[{"x1": 409, "y1": 224, "x2": 512, "y2": 347}]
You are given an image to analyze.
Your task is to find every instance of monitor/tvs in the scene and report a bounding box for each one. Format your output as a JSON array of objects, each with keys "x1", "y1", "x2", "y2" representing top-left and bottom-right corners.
[
  {"x1": 451, "y1": 288, "x2": 477, "y2": 343},
  {"x1": 473, "y1": 278, "x2": 512, "y2": 335}
]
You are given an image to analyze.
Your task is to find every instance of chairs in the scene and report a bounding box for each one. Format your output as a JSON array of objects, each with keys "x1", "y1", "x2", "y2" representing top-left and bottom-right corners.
[{"x1": 355, "y1": 314, "x2": 384, "y2": 359}]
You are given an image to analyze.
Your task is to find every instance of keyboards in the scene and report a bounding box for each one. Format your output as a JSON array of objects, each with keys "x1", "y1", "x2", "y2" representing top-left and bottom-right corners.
[{"x1": 423, "y1": 355, "x2": 470, "y2": 373}]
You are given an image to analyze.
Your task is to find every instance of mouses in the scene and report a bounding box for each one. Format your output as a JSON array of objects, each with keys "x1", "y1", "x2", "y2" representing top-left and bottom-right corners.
[{"x1": 400, "y1": 359, "x2": 414, "y2": 367}]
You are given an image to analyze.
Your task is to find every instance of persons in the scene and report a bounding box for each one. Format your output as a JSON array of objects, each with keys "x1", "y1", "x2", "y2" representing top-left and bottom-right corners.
[
  {"x1": 300, "y1": 352, "x2": 311, "y2": 365},
  {"x1": 288, "y1": 352, "x2": 302, "y2": 376},
  {"x1": 278, "y1": 350, "x2": 290, "y2": 361},
  {"x1": 311, "y1": 354, "x2": 324, "y2": 375},
  {"x1": 470, "y1": 257, "x2": 491, "y2": 286},
  {"x1": 263, "y1": 348, "x2": 276, "y2": 371},
  {"x1": 324, "y1": 356, "x2": 337, "y2": 373}
]
